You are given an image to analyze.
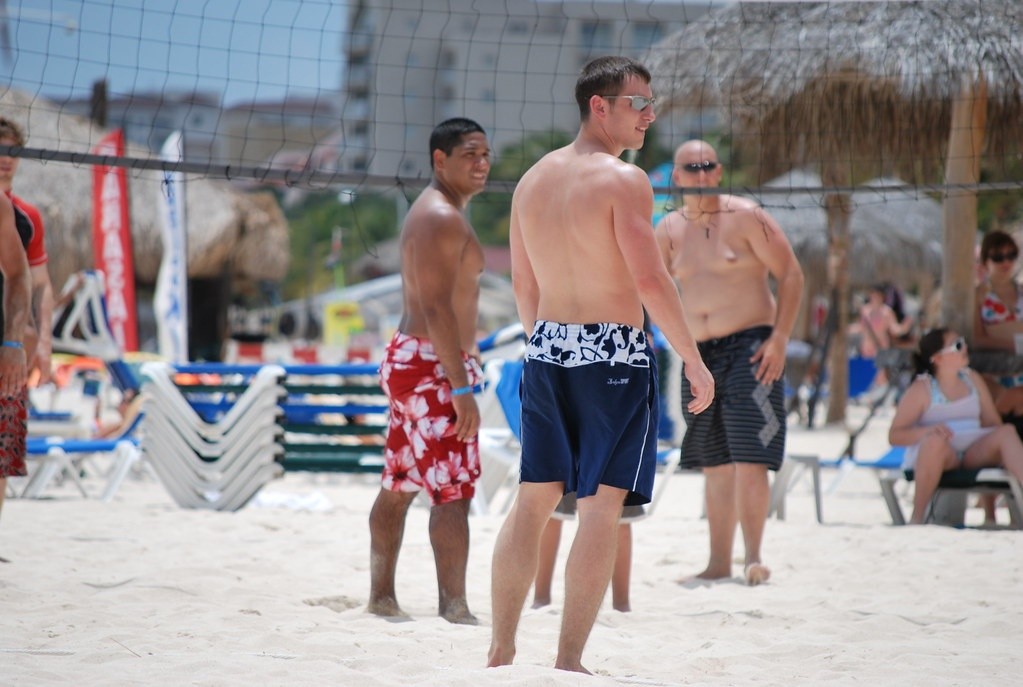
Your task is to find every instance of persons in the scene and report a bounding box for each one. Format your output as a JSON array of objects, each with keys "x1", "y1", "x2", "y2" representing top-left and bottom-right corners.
[
  {"x1": 654, "y1": 139, "x2": 805, "y2": 587},
  {"x1": 890, "y1": 326, "x2": 1023, "y2": 524},
  {"x1": 971, "y1": 231, "x2": 1023, "y2": 417},
  {"x1": 908, "y1": 298, "x2": 944, "y2": 382},
  {"x1": 848, "y1": 285, "x2": 913, "y2": 385},
  {"x1": 365, "y1": 117, "x2": 491, "y2": 625},
  {"x1": 0, "y1": 117, "x2": 55, "y2": 511},
  {"x1": 485, "y1": 57, "x2": 715, "y2": 675},
  {"x1": 0, "y1": 189, "x2": 34, "y2": 397}
]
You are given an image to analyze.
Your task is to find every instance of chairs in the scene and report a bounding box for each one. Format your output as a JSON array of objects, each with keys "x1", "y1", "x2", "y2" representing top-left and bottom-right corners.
[
  {"x1": 756, "y1": 445, "x2": 913, "y2": 525},
  {"x1": 15, "y1": 393, "x2": 143, "y2": 500}
]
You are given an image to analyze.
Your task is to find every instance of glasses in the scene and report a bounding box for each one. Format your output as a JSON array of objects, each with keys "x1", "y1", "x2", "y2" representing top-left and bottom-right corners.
[
  {"x1": 937, "y1": 336, "x2": 967, "y2": 356},
  {"x1": 601, "y1": 96, "x2": 655, "y2": 111},
  {"x1": 982, "y1": 249, "x2": 1019, "y2": 264},
  {"x1": 676, "y1": 160, "x2": 718, "y2": 172}
]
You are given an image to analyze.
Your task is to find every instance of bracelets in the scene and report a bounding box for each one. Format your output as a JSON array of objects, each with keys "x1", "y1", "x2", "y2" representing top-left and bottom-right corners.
[
  {"x1": 451, "y1": 384, "x2": 471, "y2": 396},
  {"x1": 2, "y1": 340, "x2": 23, "y2": 349}
]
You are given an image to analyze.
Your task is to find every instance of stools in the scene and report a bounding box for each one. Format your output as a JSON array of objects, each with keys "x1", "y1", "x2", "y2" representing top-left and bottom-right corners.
[{"x1": 905, "y1": 467, "x2": 1023, "y2": 529}]
